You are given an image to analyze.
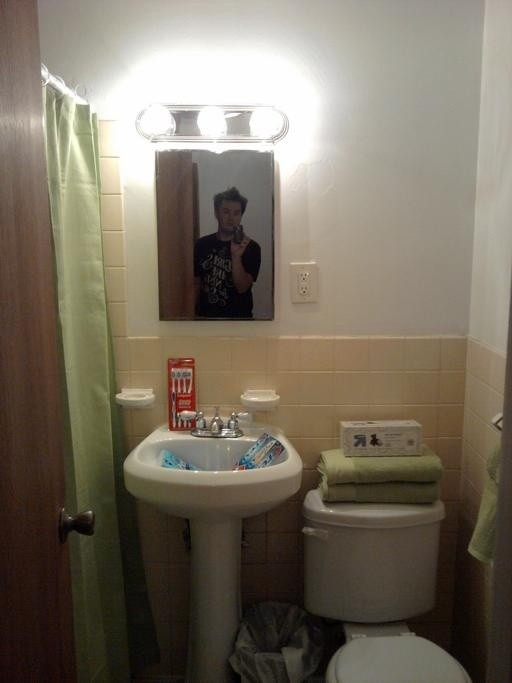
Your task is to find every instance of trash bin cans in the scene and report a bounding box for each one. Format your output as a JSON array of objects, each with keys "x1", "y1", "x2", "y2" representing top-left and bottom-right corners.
[{"x1": 236, "y1": 601, "x2": 311, "y2": 683}]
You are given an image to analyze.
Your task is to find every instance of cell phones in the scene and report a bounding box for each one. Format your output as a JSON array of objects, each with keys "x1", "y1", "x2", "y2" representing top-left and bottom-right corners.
[{"x1": 233, "y1": 225, "x2": 243, "y2": 244}]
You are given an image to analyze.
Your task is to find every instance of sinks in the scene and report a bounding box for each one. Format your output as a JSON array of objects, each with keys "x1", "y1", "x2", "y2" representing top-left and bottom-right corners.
[{"x1": 122, "y1": 419, "x2": 303, "y2": 522}]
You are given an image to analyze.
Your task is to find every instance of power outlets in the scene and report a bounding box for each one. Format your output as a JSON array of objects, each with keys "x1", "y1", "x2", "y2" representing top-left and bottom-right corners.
[{"x1": 289, "y1": 261, "x2": 319, "y2": 305}]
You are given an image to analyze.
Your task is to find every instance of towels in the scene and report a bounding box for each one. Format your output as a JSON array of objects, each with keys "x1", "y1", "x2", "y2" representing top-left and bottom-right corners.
[
  {"x1": 466, "y1": 437, "x2": 503, "y2": 565},
  {"x1": 313, "y1": 444, "x2": 444, "y2": 505}
]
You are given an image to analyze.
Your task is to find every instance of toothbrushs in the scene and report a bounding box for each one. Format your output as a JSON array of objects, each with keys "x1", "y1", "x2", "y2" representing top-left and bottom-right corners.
[{"x1": 170, "y1": 367, "x2": 194, "y2": 428}]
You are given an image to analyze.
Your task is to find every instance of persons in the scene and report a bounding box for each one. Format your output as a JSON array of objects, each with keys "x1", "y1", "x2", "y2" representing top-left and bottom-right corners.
[{"x1": 193, "y1": 186, "x2": 260, "y2": 319}]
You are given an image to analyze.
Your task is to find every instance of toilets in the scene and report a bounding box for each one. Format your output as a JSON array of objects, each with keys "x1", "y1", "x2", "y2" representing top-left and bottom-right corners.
[{"x1": 303, "y1": 487, "x2": 471, "y2": 682}]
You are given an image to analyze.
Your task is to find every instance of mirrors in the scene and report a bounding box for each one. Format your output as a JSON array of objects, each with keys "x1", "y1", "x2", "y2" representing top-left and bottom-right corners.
[{"x1": 152, "y1": 148, "x2": 276, "y2": 322}]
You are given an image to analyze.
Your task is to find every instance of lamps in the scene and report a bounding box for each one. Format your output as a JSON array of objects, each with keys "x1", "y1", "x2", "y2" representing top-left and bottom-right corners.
[{"x1": 133, "y1": 101, "x2": 290, "y2": 143}]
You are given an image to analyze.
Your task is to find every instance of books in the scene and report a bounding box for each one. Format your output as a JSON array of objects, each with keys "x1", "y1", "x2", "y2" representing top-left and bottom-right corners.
[{"x1": 157, "y1": 446, "x2": 201, "y2": 474}]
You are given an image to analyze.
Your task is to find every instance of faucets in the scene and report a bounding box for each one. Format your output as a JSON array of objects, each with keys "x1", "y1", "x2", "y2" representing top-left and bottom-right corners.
[{"x1": 209, "y1": 405, "x2": 224, "y2": 434}]
